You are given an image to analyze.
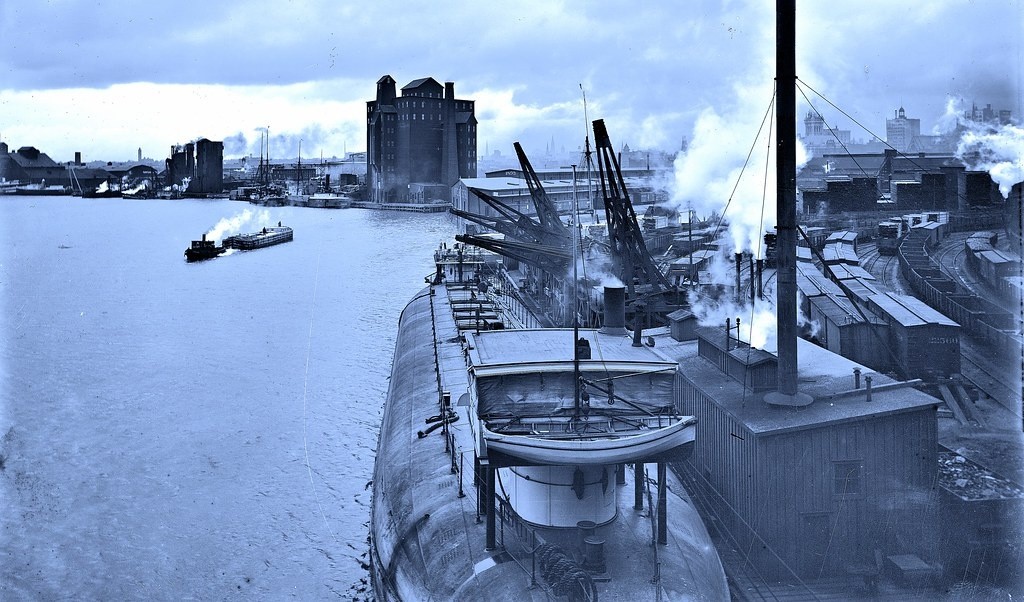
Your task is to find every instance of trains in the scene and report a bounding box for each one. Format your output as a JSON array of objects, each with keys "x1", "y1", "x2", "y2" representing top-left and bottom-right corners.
[
  {"x1": 795, "y1": 222, "x2": 1024, "y2": 390},
  {"x1": 876, "y1": 211, "x2": 948, "y2": 257}
]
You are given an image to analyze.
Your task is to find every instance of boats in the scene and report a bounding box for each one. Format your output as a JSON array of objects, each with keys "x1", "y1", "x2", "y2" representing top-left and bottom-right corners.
[
  {"x1": 0, "y1": 176, "x2": 66, "y2": 196},
  {"x1": 221, "y1": 221, "x2": 294, "y2": 251},
  {"x1": 183, "y1": 233, "x2": 226, "y2": 263},
  {"x1": 366, "y1": 237, "x2": 737, "y2": 602}
]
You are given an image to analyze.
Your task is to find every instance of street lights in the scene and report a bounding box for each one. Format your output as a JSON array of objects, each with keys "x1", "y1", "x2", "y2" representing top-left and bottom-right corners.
[
  {"x1": 297, "y1": 139, "x2": 305, "y2": 194},
  {"x1": 255, "y1": 127, "x2": 264, "y2": 184},
  {"x1": 578, "y1": 83, "x2": 597, "y2": 211},
  {"x1": 266, "y1": 126, "x2": 269, "y2": 182}
]
